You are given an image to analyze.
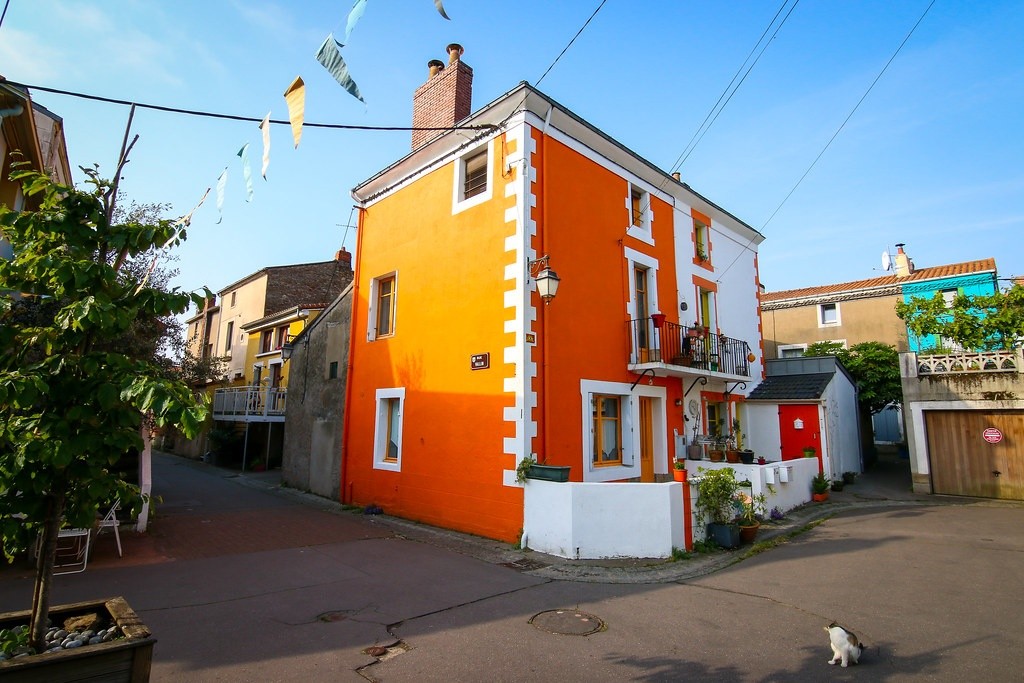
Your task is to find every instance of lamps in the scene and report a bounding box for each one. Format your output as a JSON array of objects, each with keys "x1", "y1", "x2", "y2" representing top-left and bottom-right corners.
[
  {"x1": 279, "y1": 335, "x2": 307, "y2": 363},
  {"x1": 527, "y1": 254, "x2": 561, "y2": 305},
  {"x1": 723, "y1": 381, "x2": 731, "y2": 401},
  {"x1": 676, "y1": 399, "x2": 682, "y2": 405}
]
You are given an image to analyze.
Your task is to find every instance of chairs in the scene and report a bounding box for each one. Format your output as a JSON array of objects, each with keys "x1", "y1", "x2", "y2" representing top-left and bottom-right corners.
[{"x1": 75, "y1": 498, "x2": 123, "y2": 559}]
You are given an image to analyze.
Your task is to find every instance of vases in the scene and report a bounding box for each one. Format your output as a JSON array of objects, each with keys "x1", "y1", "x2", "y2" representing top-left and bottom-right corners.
[{"x1": 672, "y1": 355, "x2": 693, "y2": 366}]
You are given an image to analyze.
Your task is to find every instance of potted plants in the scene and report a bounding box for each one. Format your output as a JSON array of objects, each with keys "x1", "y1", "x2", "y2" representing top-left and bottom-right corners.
[
  {"x1": 512, "y1": 453, "x2": 571, "y2": 485},
  {"x1": 674, "y1": 463, "x2": 688, "y2": 481},
  {"x1": 733, "y1": 493, "x2": 767, "y2": 543},
  {"x1": 741, "y1": 449, "x2": 754, "y2": 465},
  {"x1": 758, "y1": 457, "x2": 765, "y2": 465},
  {"x1": 688, "y1": 403, "x2": 704, "y2": 459},
  {"x1": 696, "y1": 466, "x2": 740, "y2": 549},
  {"x1": 803, "y1": 446, "x2": 815, "y2": 458},
  {"x1": 842, "y1": 470, "x2": 857, "y2": 484},
  {"x1": 250, "y1": 457, "x2": 265, "y2": 471},
  {"x1": 726, "y1": 418, "x2": 740, "y2": 463},
  {"x1": 812, "y1": 471, "x2": 831, "y2": 501},
  {"x1": 708, "y1": 418, "x2": 724, "y2": 463},
  {"x1": 0, "y1": 143, "x2": 155, "y2": 683}
]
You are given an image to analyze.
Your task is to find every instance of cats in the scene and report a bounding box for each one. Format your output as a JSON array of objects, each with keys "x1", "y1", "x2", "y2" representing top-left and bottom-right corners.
[{"x1": 823, "y1": 619, "x2": 863, "y2": 668}]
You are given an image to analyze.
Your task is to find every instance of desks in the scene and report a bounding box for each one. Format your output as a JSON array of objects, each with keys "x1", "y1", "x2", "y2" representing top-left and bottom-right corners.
[{"x1": 36, "y1": 523, "x2": 91, "y2": 576}]
